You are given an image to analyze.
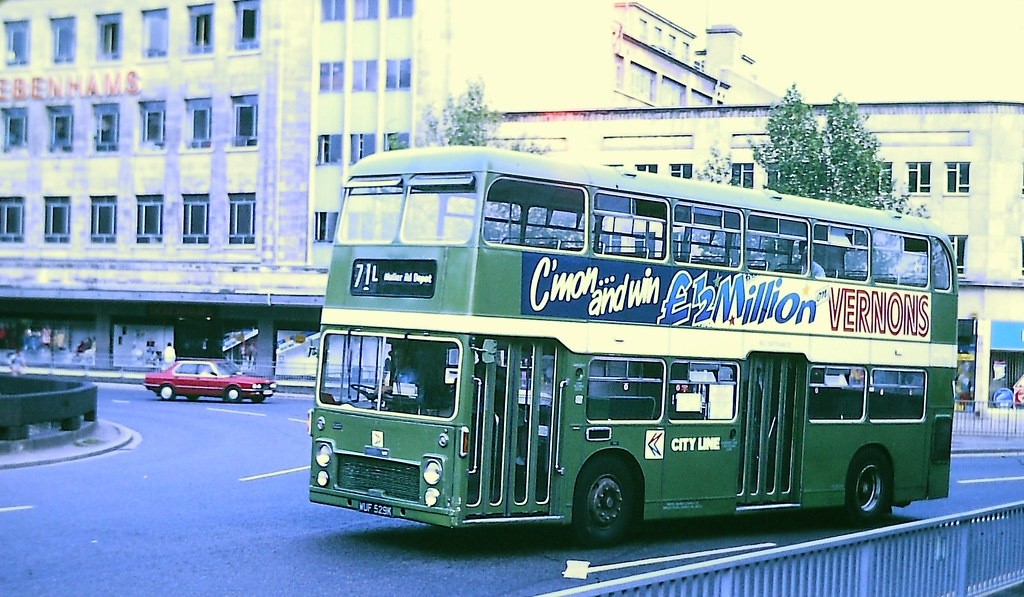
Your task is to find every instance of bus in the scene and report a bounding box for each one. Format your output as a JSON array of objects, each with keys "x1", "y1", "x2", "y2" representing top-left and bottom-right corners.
[{"x1": 309, "y1": 146, "x2": 959, "y2": 544}]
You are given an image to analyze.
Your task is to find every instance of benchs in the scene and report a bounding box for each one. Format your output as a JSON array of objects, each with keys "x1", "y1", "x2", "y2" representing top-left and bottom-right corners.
[
  {"x1": 585, "y1": 397, "x2": 659, "y2": 421},
  {"x1": 500, "y1": 237, "x2": 929, "y2": 289},
  {"x1": 809, "y1": 385, "x2": 925, "y2": 420}
]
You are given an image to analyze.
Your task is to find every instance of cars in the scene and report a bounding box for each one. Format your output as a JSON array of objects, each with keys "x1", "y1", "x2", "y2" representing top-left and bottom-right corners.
[{"x1": 145, "y1": 361, "x2": 278, "y2": 403}]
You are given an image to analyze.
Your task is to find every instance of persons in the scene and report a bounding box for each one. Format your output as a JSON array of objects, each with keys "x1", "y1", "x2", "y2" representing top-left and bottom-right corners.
[
  {"x1": 372, "y1": 344, "x2": 428, "y2": 415},
  {"x1": 162, "y1": 342, "x2": 176, "y2": 369},
  {"x1": 6, "y1": 346, "x2": 26, "y2": 375},
  {"x1": 198, "y1": 365, "x2": 216, "y2": 377},
  {"x1": 21, "y1": 324, "x2": 94, "y2": 361},
  {"x1": 796, "y1": 241, "x2": 826, "y2": 278}
]
